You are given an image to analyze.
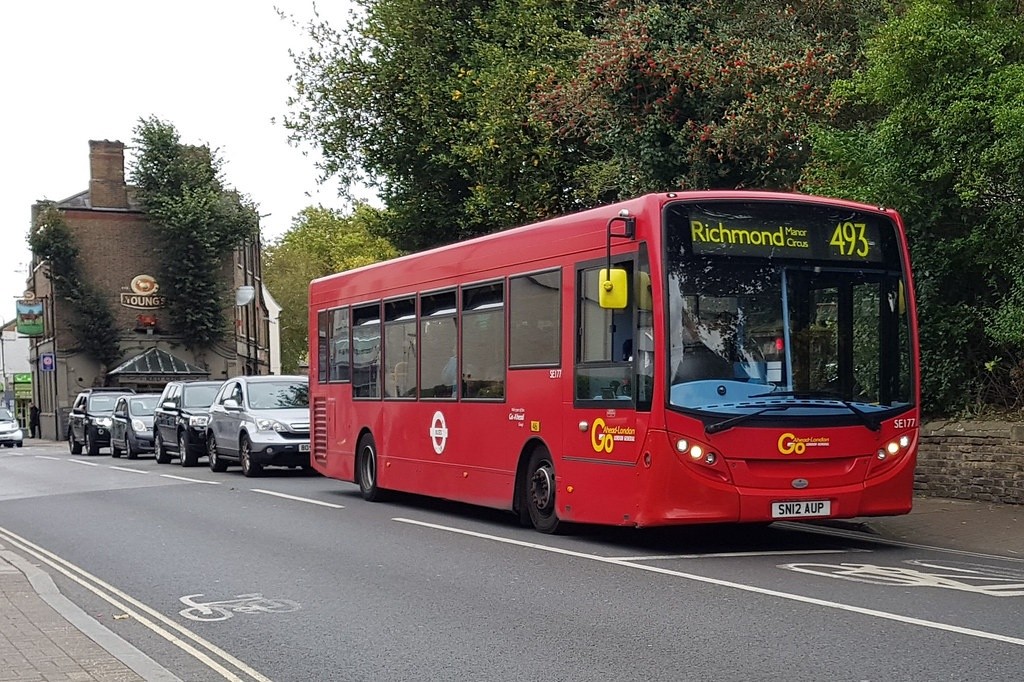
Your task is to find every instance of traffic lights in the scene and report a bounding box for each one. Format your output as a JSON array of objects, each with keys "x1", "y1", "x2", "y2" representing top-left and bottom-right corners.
[{"x1": 775, "y1": 327, "x2": 795, "y2": 365}]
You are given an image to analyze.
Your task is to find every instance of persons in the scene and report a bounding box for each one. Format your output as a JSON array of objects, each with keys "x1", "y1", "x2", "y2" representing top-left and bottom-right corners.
[
  {"x1": 441, "y1": 345, "x2": 484, "y2": 397},
  {"x1": 28, "y1": 402, "x2": 39, "y2": 439}
]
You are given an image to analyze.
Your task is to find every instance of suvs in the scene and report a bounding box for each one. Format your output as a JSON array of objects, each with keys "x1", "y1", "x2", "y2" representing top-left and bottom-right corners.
[
  {"x1": 67, "y1": 386, "x2": 148, "y2": 455},
  {"x1": 153, "y1": 380, "x2": 240, "y2": 468}
]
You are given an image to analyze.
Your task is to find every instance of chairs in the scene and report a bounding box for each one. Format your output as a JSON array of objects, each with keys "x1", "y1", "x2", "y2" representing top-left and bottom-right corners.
[
  {"x1": 132, "y1": 403, "x2": 146, "y2": 416},
  {"x1": 394, "y1": 362, "x2": 408, "y2": 398}
]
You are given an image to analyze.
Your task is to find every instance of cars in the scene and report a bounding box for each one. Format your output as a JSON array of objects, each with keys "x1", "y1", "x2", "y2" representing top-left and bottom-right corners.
[
  {"x1": 0, "y1": 407, "x2": 23, "y2": 448},
  {"x1": 205, "y1": 375, "x2": 311, "y2": 478},
  {"x1": 110, "y1": 393, "x2": 188, "y2": 460}
]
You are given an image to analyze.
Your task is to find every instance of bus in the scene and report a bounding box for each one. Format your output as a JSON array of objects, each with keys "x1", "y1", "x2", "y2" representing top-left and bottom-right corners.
[{"x1": 311, "y1": 191, "x2": 920, "y2": 534}]
